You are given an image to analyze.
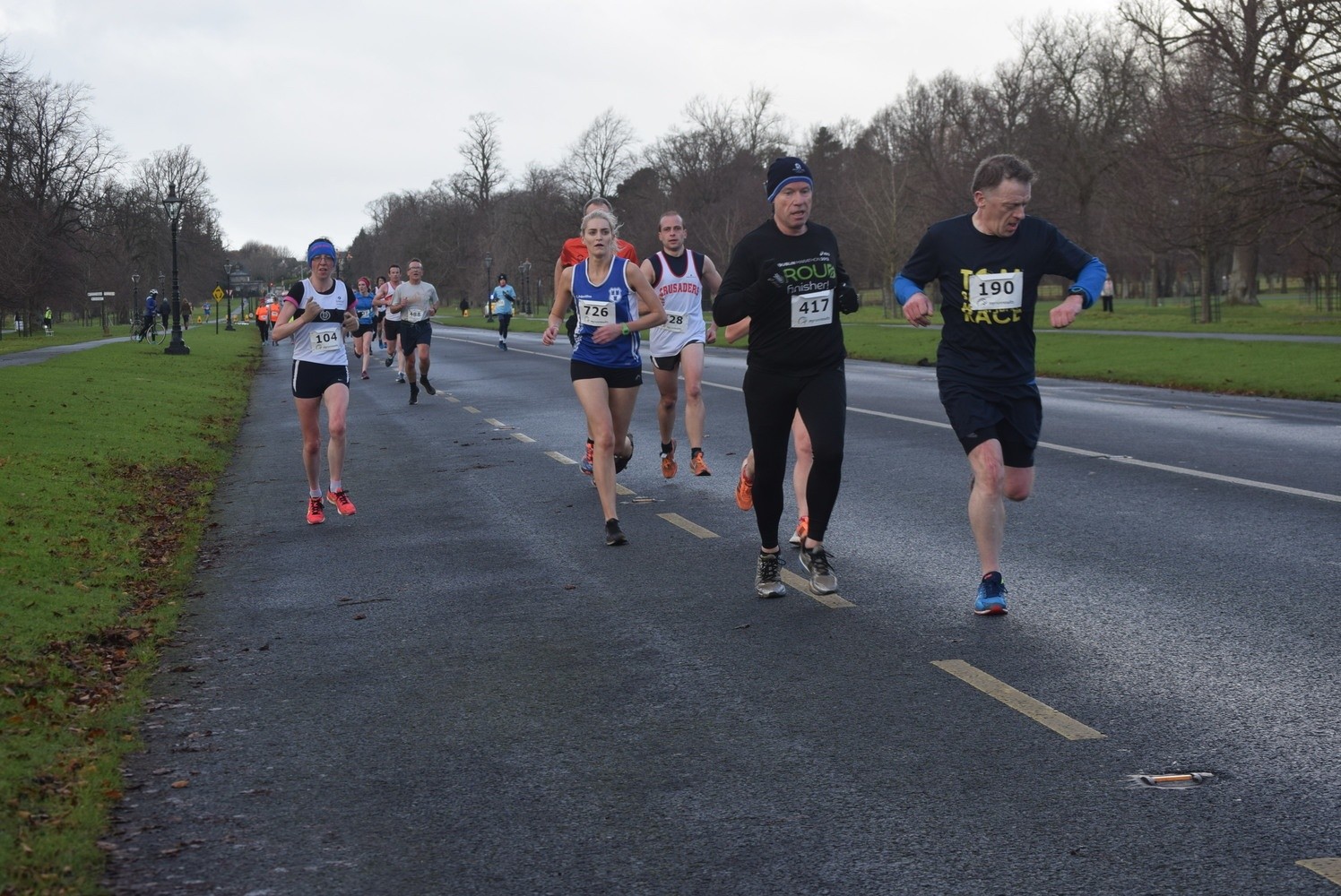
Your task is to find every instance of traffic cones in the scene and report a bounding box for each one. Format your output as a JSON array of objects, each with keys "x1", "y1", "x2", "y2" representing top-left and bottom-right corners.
[{"x1": 463, "y1": 310, "x2": 468, "y2": 317}]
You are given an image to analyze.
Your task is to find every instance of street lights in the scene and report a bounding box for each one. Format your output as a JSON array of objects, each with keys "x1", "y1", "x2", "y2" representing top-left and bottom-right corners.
[
  {"x1": 517, "y1": 257, "x2": 532, "y2": 314},
  {"x1": 157, "y1": 270, "x2": 166, "y2": 301},
  {"x1": 223, "y1": 258, "x2": 235, "y2": 330},
  {"x1": 162, "y1": 180, "x2": 191, "y2": 355},
  {"x1": 238, "y1": 279, "x2": 258, "y2": 321},
  {"x1": 131, "y1": 269, "x2": 140, "y2": 320},
  {"x1": 484, "y1": 252, "x2": 494, "y2": 321}
]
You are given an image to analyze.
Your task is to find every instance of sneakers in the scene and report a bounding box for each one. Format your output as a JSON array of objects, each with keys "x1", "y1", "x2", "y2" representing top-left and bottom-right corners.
[
  {"x1": 605, "y1": 518, "x2": 628, "y2": 545},
  {"x1": 343, "y1": 332, "x2": 436, "y2": 405},
  {"x1": 973, "y1": 571, "x2": 1007, "y2": 613},
  {"x1": 591, "y1": 478, "x2": 596, "y2": 486},
  {"x1": 735, "y1": 458, "x2": 754, "y2": 511},
  {"x1": 790, "y1": 517, "x2": 809, "y2": 546},
  {"x1": 613, "y1": 433, "x2": 634, "y2": 475},
  {"x1": 503, "y1": 343, "x2": 507, "y2": 351},
  {"x1": 660, "y1": 440, "x2": 678, "y2": 478},
  {"x1": 800, "y1": 537, "x2": 838, "y2": 595},
  {"x1": 757, "y1": 546, "x2": 786, "y2": 597},
  {"x1": 689, "y1": 451, "x2": 711, "y2": 476},
  {"x1": 579, "y1": 442, "x2": 595, "y2": 475},
  {"x1": 326, "y1": 486, "x2": 356, "y2": 517},
  {"x1": 499, "y1": 340, "x2": 504, "y2": 349},
  {"x1": 307, "y1": 496, "x2": 325, "y2": 524}
]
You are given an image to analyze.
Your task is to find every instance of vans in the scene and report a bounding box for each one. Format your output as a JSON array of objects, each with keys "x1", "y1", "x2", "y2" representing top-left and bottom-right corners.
[{"x1": 14, "y1": 315, "x2": 30, "y2": 332}]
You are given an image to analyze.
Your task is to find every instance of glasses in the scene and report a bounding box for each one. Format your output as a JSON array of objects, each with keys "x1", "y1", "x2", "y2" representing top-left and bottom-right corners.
[
  {"x1": 312, "y1": 255, "x2": 333, "y2": 262},
  {"x1": 407, "y1": 267, "x2": 421, "y2": 270}
]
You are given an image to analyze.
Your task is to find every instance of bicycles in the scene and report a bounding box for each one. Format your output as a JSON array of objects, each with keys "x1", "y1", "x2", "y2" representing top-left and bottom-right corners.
[{"x1": 129, "y1": 306, "x2": 166, "y2": 344}]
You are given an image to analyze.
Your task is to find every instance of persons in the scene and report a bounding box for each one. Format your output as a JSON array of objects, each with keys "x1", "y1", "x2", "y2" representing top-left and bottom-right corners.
[
  {"x1": 494, "y1": 273, "x2": 517, "y2": 351},
  {"x1": 540, "y1": 209, "x2": 667, "y2": 546},
  {"x1": 202, "y1": 300, "x2": 211, "y2": 324},
  {"x1": 45, "y1": 306, "x2": 52, "y2": 329},
  {"x1": 710, "y1": 157, "x2": 860, "y2": 599},
  {"x1": 256, "y1": 265, "x2": 405, "y2": 383},
  {"x1": 180, "y1": 298, "x2": 192, "y2": 329},
  {"x1": 159, "y1": 298, "x2": 171, "y2": 330},
  {"x1": 390, "y1": 258, "x2": 438, "y2": 405},
  {"x1": 1100, "y1": 273, "x2": 1116, "y2": 313},
  {"x1": 272, "y1": 237, "x2": 360, "y2": 523},
  {"x1": 725, "y1": 314, "x2": 814, "y2": 547},
  {"x1": 554, "y1": 198, "x2": 639, "y2": 473},
  {"x1": 460, "y1": 298, "x2": 468, "y2": 316},
  {"x1": 894, "y1": 155, "x2": 1106, "y2": 615},
  {"x1": 636, "y1": 211, "x2": 723, "y2": 478},
  {"x1": 138, "y1": 289, "x2": 160, "y2": 343}
]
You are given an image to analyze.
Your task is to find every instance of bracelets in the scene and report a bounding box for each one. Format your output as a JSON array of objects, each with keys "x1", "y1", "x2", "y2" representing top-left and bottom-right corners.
[{"x1": 433, "y1": 308, "x2": 436, "y2": 313}]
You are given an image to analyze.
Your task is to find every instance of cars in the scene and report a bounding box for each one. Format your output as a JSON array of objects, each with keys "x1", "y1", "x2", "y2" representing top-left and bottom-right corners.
[{"x1": 484, "y1": 295, "x2": 516, "y2": 318}]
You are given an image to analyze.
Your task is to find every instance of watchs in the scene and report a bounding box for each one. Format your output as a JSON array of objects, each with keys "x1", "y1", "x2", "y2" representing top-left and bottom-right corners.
[
  {"x1": 1069, "y1": 287, "x2": 1088, "y2": 305},
  {"x1": 620, "y1": 322, "x2": 630, "y2": 337}
]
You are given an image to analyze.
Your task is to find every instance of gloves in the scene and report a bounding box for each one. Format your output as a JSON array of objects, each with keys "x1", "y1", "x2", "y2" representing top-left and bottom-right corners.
[
  {"x1": 756, "y1": 259, "x2": 786, "y2": 302},
  {"x1": 838, "y1": 285, "x2": 859, "y2": 314}
]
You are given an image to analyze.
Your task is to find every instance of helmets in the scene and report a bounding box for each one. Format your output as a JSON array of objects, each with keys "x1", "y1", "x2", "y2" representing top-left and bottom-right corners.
[{"x1": 149, "y1": 289, "x2": 158, "y2": 297}]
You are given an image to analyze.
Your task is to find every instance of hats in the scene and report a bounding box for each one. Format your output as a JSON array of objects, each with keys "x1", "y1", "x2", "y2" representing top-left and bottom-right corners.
[
  {"x1": 498, "y1": 273, "x2": 506, "y2": 282},
  {"x1": 767, "y1": 157, "x2": 812, "y2": 202}
]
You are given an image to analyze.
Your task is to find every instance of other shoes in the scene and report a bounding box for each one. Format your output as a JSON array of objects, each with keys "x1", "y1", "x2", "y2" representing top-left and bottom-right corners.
[
  {"x1": 135, "y1": 335, "x2": 141, "y2": 341},
  {"x1": 152, "y1": 341, "x2": 158, "y2": 345},
  {"x1": 262, "y1": 339, "x2": 295, "y2": 346}
]
